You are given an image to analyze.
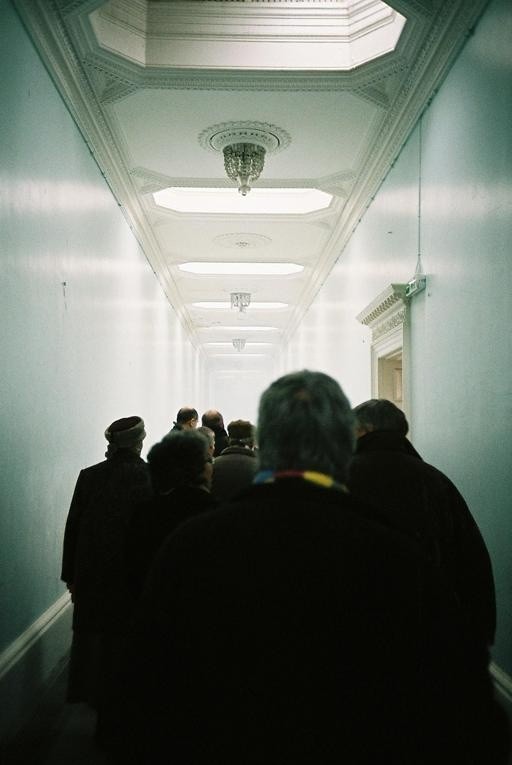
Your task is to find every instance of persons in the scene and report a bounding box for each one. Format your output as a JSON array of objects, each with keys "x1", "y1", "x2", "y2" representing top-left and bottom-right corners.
[
  {"x1": 60, "y1": 407, "x2": 259, "y2": 763},
  {"x1": 351, "y1": 398, "x2": 498, "y2": 654},
  {"x1": 138, "y1": 370, "x2": 498, "y2": 762}
]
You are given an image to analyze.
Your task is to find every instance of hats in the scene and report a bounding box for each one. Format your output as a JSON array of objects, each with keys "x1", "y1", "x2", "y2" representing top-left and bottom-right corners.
[
  {"x1": 227, "y1": 419, "x2": 255, "y2": 439},
  {"x1": 104, "y1": 417, "x2": 146, "y2": 459}
]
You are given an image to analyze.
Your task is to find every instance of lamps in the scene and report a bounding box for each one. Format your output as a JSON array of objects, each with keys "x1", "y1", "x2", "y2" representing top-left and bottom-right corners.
[
  {"x1": 229, "y1": 292, "x2": 252, "y2": 313},
  {"x1": 197, "y1": 120, "x2": 292, "y2": 198}
]
